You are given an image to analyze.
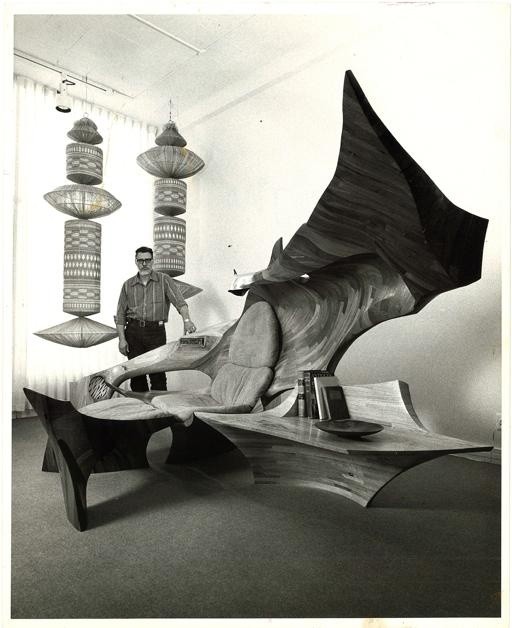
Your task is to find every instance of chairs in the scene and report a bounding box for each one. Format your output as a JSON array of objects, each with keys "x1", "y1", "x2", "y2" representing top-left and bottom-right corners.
[
  {"x1": 149, "y1": 299, "x2": 282, "y2": 485},
  {"x1": 19, "y1": 382, "x2": 176, "y2": 534}
]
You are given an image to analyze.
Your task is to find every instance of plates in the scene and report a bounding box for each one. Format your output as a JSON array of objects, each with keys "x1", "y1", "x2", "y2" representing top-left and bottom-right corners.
[{"x1": 312, "y1": 418, "x2": 384, "y2": 440}]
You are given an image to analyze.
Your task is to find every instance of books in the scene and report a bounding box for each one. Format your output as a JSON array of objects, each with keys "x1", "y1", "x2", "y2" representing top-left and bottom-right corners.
[{"x1": 297, "y1": 370, "x2": 351, "y2": 421}]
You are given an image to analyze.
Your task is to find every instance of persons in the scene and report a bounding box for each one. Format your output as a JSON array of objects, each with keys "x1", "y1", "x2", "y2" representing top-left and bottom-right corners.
[{"x1": 113, "y1": 247, "x2": 196, "y2": 392}]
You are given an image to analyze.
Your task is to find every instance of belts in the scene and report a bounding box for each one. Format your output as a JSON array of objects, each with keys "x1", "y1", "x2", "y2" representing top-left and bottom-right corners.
[{"x1": 127, "y1": 319, "x2": 163, "y2": 329}]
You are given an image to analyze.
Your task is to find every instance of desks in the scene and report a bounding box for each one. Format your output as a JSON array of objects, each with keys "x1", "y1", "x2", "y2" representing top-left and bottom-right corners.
[{"x1": 189, "y1": 374, "x2": 497, "y2": 513}]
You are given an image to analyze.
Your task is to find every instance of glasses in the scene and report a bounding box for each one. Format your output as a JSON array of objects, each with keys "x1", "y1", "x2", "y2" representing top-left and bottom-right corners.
[{"x1": 135, "y1": 257, "x2": 152, "y2": 263}]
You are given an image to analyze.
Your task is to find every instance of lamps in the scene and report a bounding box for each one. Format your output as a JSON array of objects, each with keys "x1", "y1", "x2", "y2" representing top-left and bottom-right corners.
[
  {"x1": 30, "y1": 69, "x2": 122, "y2": 353},
  {"x1": 131, "y1": 95, "x2": 205, "y2": 306}
]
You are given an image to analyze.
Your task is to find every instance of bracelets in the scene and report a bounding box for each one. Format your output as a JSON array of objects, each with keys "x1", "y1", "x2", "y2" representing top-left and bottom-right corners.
[{"x1": 183, "y1": 319, "x2": 192, "y2": 323}]
